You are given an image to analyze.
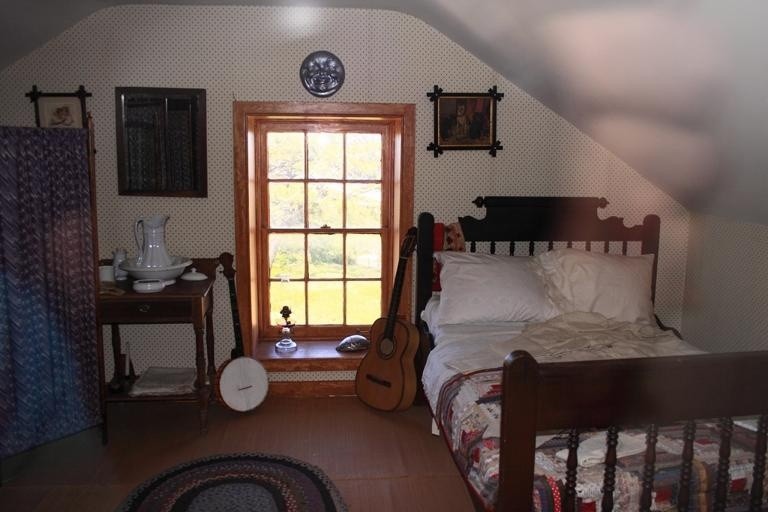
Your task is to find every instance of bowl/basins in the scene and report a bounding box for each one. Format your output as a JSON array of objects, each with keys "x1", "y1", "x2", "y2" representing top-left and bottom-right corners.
[{"x1": 118, "y1": 256, "x2": 195, "y2": 287}]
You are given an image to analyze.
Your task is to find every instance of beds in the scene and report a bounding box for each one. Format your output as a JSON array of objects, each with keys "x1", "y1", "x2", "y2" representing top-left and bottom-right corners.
[{"x1": 411, "y1": 194, "x2": 766, "y2": 512}]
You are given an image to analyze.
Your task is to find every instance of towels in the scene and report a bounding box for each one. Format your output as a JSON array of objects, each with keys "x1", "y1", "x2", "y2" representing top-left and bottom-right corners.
[{"x1": 557, "y1": 430, "x2": 647, "y2": 469}]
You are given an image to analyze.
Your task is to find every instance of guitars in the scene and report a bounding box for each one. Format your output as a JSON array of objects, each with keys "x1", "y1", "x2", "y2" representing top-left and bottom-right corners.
[{"x1": 355, "y1": 227, "x2": 421, "y2": 413}]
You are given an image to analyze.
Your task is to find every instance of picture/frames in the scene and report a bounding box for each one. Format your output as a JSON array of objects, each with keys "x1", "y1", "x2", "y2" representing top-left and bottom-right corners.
[
  {"x1": 423, "y1": 84, "x2": 505, "y2": 159},
  {"x1": 24, "y1": 85, "x2": 93, "y2": 128}
]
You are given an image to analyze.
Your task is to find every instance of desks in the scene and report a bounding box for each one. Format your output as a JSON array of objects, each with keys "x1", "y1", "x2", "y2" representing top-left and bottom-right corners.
[{"x1": 98, "y1": 258, "x2": 219, "y2": 445}]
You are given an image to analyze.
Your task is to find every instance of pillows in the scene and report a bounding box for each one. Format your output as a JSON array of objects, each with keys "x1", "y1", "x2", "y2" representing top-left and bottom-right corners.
[{"x1": 436, "y1": 248, "x2": 658, "y2": 328}]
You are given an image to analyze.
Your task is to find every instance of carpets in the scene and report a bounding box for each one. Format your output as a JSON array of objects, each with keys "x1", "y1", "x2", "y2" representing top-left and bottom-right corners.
[{"x1": 115, "y1": 452, "x2": 345, "y2": 512}]
